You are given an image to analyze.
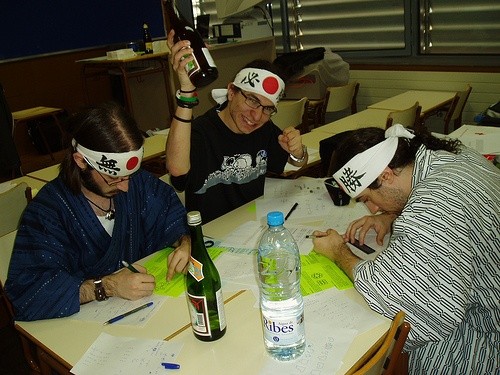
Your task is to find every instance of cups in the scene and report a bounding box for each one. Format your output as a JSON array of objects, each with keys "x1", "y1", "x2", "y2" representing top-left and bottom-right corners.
[{"x1": 324, "y1": 179, "x2": 350, "y2": 206}]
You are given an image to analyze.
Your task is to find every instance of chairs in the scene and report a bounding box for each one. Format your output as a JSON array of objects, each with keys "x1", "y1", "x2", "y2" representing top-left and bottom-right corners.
[{"x1": 0, "y1": 80, "x2": 473, "y2": 375}]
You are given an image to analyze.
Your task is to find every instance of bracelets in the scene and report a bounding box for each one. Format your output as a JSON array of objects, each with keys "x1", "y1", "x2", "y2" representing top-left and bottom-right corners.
[
  {"x1": 94, "y1": 277, "x2": 108, "y2": 302},
  {"x1": 174, "y1": 87, "x2": 199, "y2": 123}
]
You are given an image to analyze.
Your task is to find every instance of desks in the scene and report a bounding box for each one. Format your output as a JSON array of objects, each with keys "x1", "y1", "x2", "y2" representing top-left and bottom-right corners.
[
  {"x1": 366, "y1": 89, "x2": 457, "y2": 114},
  {"x1": 266, "y1": 131, "x2": 338, "y2": 176},
  {"x1": 76, "y1": 36, "x2": 277, "y2": 137},
  {"x1": 14, "y1": 176, "x2": 393, "y2": 375},
  {"x1": 448, "y1": 101, "x2": 500, "y2": 155},
  {"x1": 311, "y1": 109, "x2": 401, "y2": 134},
  {"x1": 12, "y1": 106, "x2": 68, "y2": 161},
  {"x1": 26, "y1": 133, "x2": 168, "y2": 183}
]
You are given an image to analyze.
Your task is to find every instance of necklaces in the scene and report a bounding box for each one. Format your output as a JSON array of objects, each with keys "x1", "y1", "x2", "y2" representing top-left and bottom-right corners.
[{"x1": 86, "y1": 195, "x2": 115, "y2": 221}]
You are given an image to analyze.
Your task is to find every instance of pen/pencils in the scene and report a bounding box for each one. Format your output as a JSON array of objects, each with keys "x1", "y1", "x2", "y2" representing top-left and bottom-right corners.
[
  {"x1": 162, "y1": 363, "x2": 180, "y2": 369},
  {"x1": 306, "y1": 235, "x2": 324, "y2": 238},
  {"x1": 285, "y1": 203, "x2": 298, "y2": 220},
  {"x1": 121, "y1": 260, "x2": 140, "y2": 273},
  {"x1": 104, "y1": 302, "x2": 153, "y2": 326}
]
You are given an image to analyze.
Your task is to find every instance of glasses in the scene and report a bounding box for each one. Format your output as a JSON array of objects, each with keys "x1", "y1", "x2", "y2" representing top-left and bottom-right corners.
[
  {"x1": 239, "y1": 88, "x2": 278, "y2": 115},
  {"x1": 94, "y1": 168, "x2": 138, "y2": 187}
]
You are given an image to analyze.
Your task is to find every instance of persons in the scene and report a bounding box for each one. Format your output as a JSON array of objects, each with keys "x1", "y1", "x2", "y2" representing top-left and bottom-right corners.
[
  {"x1": 167, "y1": 29, "x2": 309, "y2": 225},
  {"x1": 5, "y1": 103, "x2": 192, "y2": 321},
  {"x1": 312, "y1": 126, "x2": 500, "y2": 375}
]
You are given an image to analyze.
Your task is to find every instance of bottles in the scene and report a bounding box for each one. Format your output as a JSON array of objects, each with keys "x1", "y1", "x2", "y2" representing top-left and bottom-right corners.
[
  {"x1": 258, "y1": 211, "x2": 305, "y2": 361},
  {"x1": 164, "y1": 0, "x2": 219, "y2": 88},
  {"x1": 128, "y1": 40, "x2": 138, "y2": 53},
  {"x1": 186, "y1": 211, "x2": 227, "y2": 342},
  {"x1": 142, "y1": 24, "x2": 154, "y2": 53}
]
